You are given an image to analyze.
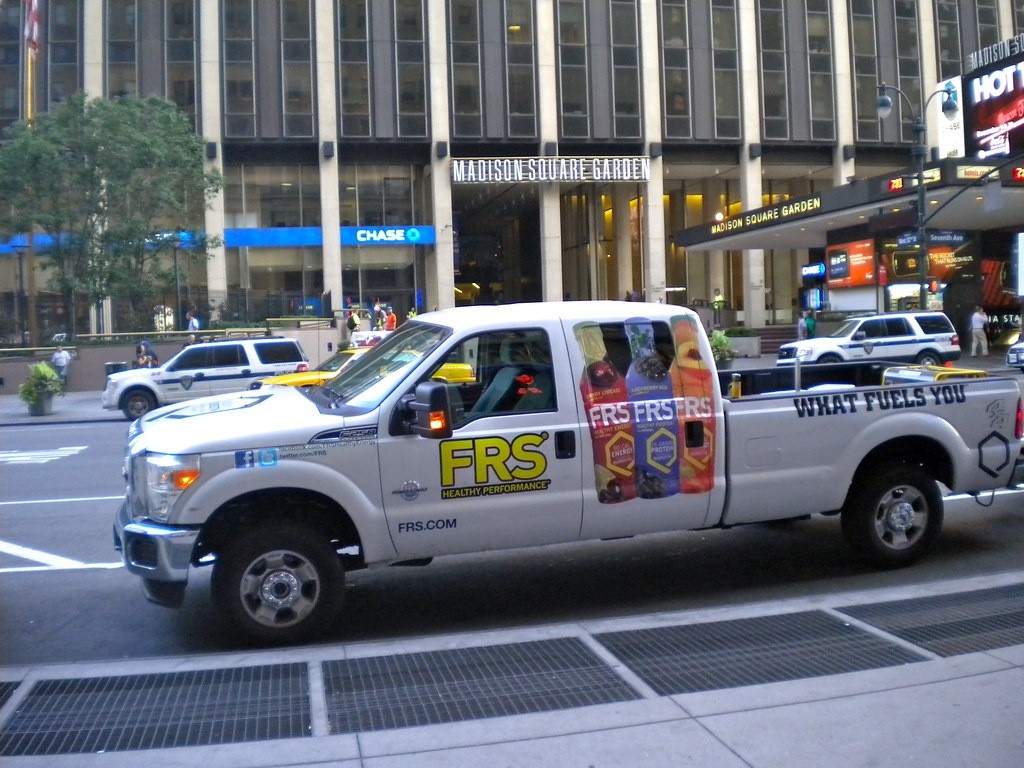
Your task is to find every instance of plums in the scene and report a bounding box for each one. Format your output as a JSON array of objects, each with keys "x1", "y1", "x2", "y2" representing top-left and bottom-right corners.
[{"x1": 587, "y1": 360, "x2": 618, "y2": 386}]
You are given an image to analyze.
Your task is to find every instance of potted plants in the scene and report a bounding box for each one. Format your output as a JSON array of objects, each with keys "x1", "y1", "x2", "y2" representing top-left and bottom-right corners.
[
  {"x1": 709, "y1": 329, "x2": 739, "y2": 370},
  {"x1": 18, "y1": 361, "x2": 64, "y2": 417},
  {"x1": 726, "y1": 328, "x2": 761, "y2": 358}
]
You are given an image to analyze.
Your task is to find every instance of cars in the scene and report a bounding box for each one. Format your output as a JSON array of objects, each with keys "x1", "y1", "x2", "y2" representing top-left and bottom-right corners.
[
  {"x1": 1006, "y1": 342, "x2": 1024, "y2": 373},
  {"x1": 256, "y1": 346, "x2": 479, "y2": 387}
]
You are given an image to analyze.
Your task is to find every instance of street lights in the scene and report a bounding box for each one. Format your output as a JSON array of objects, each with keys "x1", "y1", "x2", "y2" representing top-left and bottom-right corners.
[{"x1": 875, "y1": 81, "x2": 961, "y2": 314}]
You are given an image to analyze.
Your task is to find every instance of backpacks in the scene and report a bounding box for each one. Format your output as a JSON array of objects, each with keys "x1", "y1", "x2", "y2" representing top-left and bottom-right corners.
[{"x1": 347, "y1": 315, "x2": 357, "y2": 330}]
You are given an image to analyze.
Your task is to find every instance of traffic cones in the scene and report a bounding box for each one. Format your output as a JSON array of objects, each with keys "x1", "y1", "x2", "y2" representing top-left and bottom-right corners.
[{"x1": 945, "y1": 359, "x2": 953, "y2": 367}]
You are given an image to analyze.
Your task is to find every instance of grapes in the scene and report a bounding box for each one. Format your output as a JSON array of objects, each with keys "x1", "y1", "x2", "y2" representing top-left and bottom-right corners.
[{"x1": 635, "y1": 357, "x2": 666, "y2": 380}]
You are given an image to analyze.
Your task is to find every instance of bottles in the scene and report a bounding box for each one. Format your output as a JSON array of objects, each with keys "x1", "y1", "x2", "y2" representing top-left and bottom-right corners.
[
  {"x1": 573, "y1": 321, "x2": 635, "y2": 504},
  {"x1": 668, "y1": 315, "x2": 716, "y2": 494},
  {"x1": 732, "y1": 373, "x2": 742, "y2": 398},
  {"x1": 624, "y1": 317, "x2": 681, "y2": 500}
]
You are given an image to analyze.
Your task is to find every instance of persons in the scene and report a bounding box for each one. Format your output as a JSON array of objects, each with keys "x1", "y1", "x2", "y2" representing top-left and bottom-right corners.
[
  {"x1": 797, "y1": 309, "x2": 816, "y2": 340},
  {"x1": 971, "y1": 307, "x2": 988, "y2": 356},
  {"x1": 186, "y1": 311, "x2": 198, "y2": 337},
  {"x1": 713, "y1": 288, "x2": 724, "y2": 310},
  {"x1": 52, "y1": 345, "x2": 70, "y2": 395},
  {"x1": 349, "y1": 311, "x2": 361, "y2": 332},
  {"x1": 406, "y1": 307, "x2": 416, "y2": 318},
  {"x1": 136, "y1": 340, "x2": 158, "y2": 368},
  {"x1": 373, "y1": 306, "x2": 396, "y2": 331}
]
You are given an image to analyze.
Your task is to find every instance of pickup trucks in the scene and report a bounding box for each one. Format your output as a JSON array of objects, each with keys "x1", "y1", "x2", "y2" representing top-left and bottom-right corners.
[{"x1": 109, "y1": 298, "x2": 1024, "y2": 645}]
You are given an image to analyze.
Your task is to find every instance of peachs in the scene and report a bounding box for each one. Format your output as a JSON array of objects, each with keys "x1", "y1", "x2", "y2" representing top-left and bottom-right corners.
[{"x1": 677, "y1": 342, "x2": 711, "y2": 379}]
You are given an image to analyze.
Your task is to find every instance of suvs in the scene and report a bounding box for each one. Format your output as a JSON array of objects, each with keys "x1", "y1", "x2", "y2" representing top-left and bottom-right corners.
[
  {"x1": 776, "y1": 309, "x2": 962, "y2": 368},
  {"x1": 100, "y1": 336, "x2": 309, "y2": 421}
]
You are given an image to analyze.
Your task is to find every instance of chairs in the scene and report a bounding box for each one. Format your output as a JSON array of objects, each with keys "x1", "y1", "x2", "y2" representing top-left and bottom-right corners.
[{"x1": 466, "y1": 364, "x2": 553, "y2": 414}]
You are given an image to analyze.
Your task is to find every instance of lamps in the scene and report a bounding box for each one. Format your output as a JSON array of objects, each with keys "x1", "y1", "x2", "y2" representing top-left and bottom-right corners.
[
  {"x1": 205, "y1": 141, "x2": 216, "y2": 159},
  {"x1": 546, "y1": 142, "x2": 557, "y2": 156},
  {"x1": 323, "y1": 141, "x2": 334, "y2": 158},
  {"x1": 750, "y1": 144, "x2": 762, "y2": 159},
  {"x1": 436, "y1": 142, "x2": 447, "y2": 159},
  {"x1": 843, "y1": 144, "x2": 855, "y2": 160},
  {"x1": 649, "y1": 142, "x2": 661, "y2": 158}
]
